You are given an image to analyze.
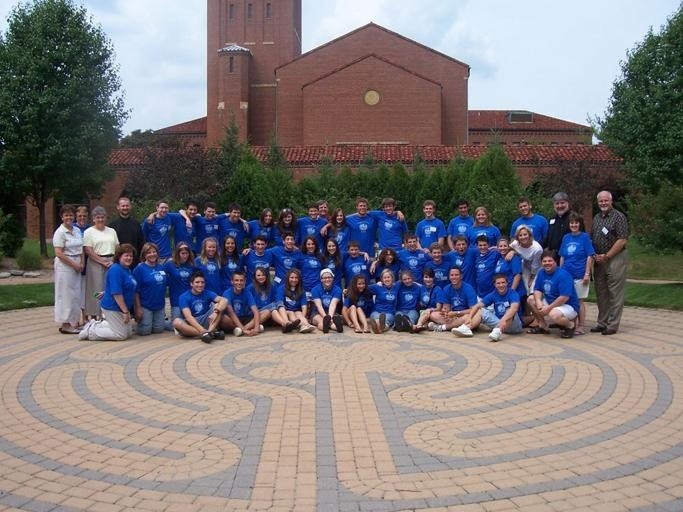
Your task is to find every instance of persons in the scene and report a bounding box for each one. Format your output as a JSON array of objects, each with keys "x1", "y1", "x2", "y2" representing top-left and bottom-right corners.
[
  {"x1": 51, "y1": 190, "x2": 595, "y2": 342},
  {"x1": 589, "y1": 190, "x2": 629, "y2": 336}
]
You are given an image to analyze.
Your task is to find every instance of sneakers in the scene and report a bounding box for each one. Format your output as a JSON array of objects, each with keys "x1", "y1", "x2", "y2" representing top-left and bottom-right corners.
[
  {"x1": 527, "y1": 327, "x2": 549, "y2": 334},
  {"x1": 591, "y1": 325, "x2": 603, "y2": 332},
  {"x1": 489, "y1": 328, "x2": 501, "y2": 341},
  {"x1": 354, "y1": 313, "x2": 443, "y2": 333},
  {"x1": 451, "y1": 324, "x2": 473, "y2": 337},
  {"x1": 562, "y1": 324, "x2": 576, "y2": 338},
  {"x1": 602, "y1": 327, "x2": 616, "y2": 334},
  {"x1": 59, "y1": 326, "x2": 80, "y2": 334},
  {"x1": 80, "y1": 319, "x2": 97, "y2": 340},
  {"x1": 202, "y1": 314, "x2": 343, "y2": 342}
]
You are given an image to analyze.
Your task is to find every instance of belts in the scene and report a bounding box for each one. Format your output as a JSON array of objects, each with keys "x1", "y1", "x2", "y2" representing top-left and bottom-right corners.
[{"x1": 98, "y1": 254, "x2": 114, "y2": 257}]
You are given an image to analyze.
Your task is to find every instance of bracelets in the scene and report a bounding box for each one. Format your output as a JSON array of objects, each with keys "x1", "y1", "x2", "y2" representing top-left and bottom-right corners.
[{"x1": 603, "y1": 253, "x2": 609, "y2": 261}]
